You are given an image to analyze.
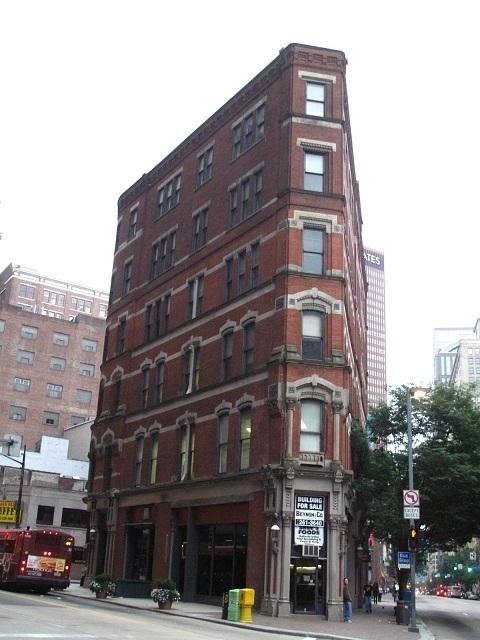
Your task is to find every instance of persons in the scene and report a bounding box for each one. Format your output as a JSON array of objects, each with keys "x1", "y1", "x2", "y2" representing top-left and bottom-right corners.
[
  {"x1": 363, "y1": 583, "x2": 372, "y2": 613},
  {"x1": 344, "y1": 578, "x2": 353, "y2": 623},
  {"x1": 378, "y1": 585, "x2": 384, "y2": 602},
  {"x1": 80, "y1": 560, "x2": 87, "y2": 587},
  {"x1": 373, "y1": 582, "x2": 377, "y2": 604}
]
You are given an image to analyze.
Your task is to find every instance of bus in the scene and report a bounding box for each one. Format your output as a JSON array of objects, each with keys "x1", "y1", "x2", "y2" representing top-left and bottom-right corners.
[{"x1": 0, "y1": 526, "x2": 76, "y2": 596}]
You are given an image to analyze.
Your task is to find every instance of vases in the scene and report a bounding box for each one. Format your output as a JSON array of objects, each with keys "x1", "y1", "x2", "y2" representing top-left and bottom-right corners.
[
  {"x1": 96, "y1": 590, "x2": 109, "y2": 599},
  {"x1": 159, "y1": 598, "x2": 172, "y2": 609}
]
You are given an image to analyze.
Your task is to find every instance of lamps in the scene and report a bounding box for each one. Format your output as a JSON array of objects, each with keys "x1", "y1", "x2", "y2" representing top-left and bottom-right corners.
[
  {"x1": 270, "y1": 524, "x2": 280, "y2": 538},
  {"x1": 356, "y1": 543, "x2": 364, "y2": 551}
]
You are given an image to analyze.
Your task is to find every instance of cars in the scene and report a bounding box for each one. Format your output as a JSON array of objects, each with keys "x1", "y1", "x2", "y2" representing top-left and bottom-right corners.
[{"x1": 421, "y1": 585, "x2": 479, "y2": 600}]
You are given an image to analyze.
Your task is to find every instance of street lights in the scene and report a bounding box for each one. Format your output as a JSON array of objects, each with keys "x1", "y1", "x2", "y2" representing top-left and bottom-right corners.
[{"x1": 402, "y1": 386, "x2": 434, "y2": 631}]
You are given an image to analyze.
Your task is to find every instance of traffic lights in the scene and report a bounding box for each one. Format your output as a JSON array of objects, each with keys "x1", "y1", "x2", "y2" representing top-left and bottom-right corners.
[{"x1": 409, "y1": 528, "x2": 418, "y2": 539}]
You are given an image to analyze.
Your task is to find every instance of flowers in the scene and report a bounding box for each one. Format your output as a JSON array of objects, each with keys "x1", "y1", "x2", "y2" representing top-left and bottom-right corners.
[
  {"x1": 88, "y1": 572, "x2": 116, "y2": 595},
  {"x1": 150, "y1": 580, "x2": 181, "y2": 603}
]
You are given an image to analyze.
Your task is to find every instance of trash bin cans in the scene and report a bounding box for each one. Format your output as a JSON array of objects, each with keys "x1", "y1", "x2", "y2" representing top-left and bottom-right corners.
[{"x1": 394, "y1": 590, "x2": 412, "y2": 626}]
